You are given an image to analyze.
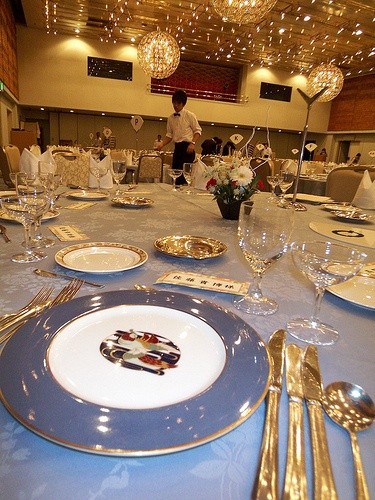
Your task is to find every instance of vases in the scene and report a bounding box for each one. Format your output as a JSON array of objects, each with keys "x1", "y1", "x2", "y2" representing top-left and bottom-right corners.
[{"x1": 216, "y1": 203, "x2": 250, "y2": 220}]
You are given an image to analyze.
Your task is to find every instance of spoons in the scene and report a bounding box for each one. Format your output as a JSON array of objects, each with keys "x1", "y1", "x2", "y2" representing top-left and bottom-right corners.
[
  {"x1": 322, "y1": 380, "x2": 375, "y2": 500},
  {"x1": 0, "y1": 224, "x2": 10, "y2": 242}
]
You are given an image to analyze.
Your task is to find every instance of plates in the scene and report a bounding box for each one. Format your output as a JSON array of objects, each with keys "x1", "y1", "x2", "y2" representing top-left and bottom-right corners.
[
  {"x1": 54, "y1": 241, "x2": 148, "y2": 275},
  {"x1": 319, "y1": 260, "x2": 375, "y2": 311},
  {"x1": 0, "y1": 185, "x2": 110, "y2": 224},
  {"x1": 323, "y1": 202, "x2": 374, "y2": 222},
  {"x1": 154, "y1": 234, "x2": 228, "y2": 259},
  {"x1": 111, "y1": 197, "x2": 154, "y2": 208},
  {"x1": 0, "y1": 290, "x2": 273, "y2": 457}
]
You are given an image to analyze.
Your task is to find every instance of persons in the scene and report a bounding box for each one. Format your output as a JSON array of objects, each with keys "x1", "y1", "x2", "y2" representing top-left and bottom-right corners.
[
  {"x1": 349, "y1": 153, "x2": 361, "y2": 167},
  {"x1": 90, "y1": 132, "x2": 102, "y2": 148},
  {"x1": 320, "y1": 148, "x2": 328, "y2": 162},
  {"x1": 154, "y1": 135, "x2": 163, "y2": 150},
  {"x1": 262, "y1": 144, "x2": 273, "y2": 157},
  {"x1": 157, "y1": 90, "x2": 202, "y2": 185},
  {"x1": 201, "y1": 137, "x2": 235, "y2": 155}
]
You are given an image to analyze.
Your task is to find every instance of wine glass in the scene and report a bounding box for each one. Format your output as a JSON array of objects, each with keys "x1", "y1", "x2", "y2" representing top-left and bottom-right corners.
[
  {"x1": 267, "y1": 176, "x2": 280, "y2": 202},
  {"x1": 275, "y1": 169, "x2": 294, "y2": 203},
  {"x1": 286, "y1": 240, "x2": 366, "y2": 345},
  {"x1": 4, "y1": 158, "x2": 63, "y2": 263},
  {"x1": 110, "y1": 160, "x2": 127, "y2": 196},
  {"x1": 168, "y1": 169, "x2": 183, "y2": 191},
  {"x1": 183, "y1": 163, "x2": 195, "y2": 193},
  {"x1": 232, "y1": 199, "x2": 294, "y2": 316},
  {"x1": 89, "y1": 167, "x2": 108, "y2": 193}
]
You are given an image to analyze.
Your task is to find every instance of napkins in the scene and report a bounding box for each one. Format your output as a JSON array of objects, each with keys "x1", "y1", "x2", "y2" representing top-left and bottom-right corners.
[{"x1": 284, "y1": 193, "x2": 335, "y2": 205}]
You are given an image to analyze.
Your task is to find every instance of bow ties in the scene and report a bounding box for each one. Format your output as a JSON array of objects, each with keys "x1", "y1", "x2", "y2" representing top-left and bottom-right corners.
[{"x1": 173, "y1": 113, "x2": 180, "y2": 117}]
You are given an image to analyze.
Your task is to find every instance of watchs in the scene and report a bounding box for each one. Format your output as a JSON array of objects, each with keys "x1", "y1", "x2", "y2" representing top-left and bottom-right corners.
[{"x1": 191, "y1": 141, "x2": 196, "y2": 145}]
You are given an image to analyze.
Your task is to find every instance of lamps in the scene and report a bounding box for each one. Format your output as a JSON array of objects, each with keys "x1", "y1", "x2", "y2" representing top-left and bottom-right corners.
[{"x1": 136, "y1": 0, "x2": 343, "y2": 102}]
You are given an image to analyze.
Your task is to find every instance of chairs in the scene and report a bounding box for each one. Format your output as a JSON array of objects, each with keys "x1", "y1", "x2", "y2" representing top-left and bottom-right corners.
[{"x1": 0, "y1": 144, "x2": 375, "y2": 210}]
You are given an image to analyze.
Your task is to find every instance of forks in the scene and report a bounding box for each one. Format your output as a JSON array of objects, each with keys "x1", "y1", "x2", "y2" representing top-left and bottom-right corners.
[{"x1": 0, "y1": 277, "x2": 85, "y2": 345}]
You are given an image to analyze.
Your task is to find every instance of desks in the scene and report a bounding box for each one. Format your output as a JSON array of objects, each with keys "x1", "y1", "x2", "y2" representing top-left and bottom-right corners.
[{"x1": 0, "y1": 183, "x2": 375, "y2": 500}]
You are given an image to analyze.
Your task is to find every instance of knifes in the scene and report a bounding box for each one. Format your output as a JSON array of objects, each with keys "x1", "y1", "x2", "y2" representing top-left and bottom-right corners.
[
  {"x1": 300, "y1": 344, "x2": 338, "y2": 500},
  {"x1": 282, "y1": 343, "x2": 309, "y2": 499},
  {"x1": 252, "y1": 329, "x2": 286, "y2": 500},
  {"x1": 34, "y1": 270, "x2": 100, "y2": 288}
]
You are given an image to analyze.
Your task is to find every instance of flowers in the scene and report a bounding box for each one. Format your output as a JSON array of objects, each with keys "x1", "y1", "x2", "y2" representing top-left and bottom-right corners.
[{"x1": 205, "y1": 157, "x2": 264, "y2": 207}]
